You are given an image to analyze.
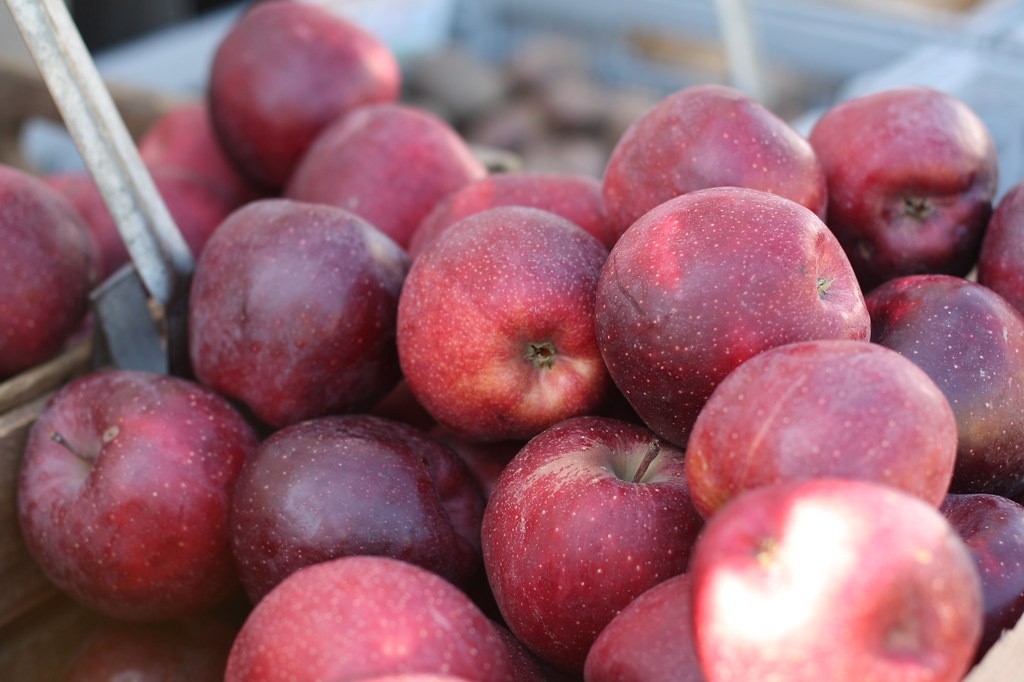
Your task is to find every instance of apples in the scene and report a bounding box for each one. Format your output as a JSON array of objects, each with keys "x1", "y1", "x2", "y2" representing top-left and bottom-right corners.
[{"x1": 0, "y1": 0, "x2": 1024, "y2": 682}]
